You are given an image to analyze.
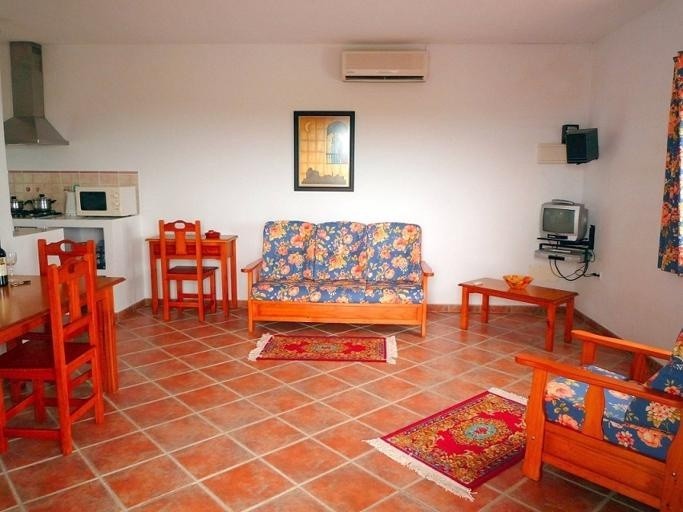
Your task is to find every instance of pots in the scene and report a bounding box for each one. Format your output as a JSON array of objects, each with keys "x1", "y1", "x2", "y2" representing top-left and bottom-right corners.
[
  {"x1": 25, "y1": 194, "x2": 57, "y2": 210},
  {"x1": 11, "y1": 195, "x2": 27, "y2": 212}
]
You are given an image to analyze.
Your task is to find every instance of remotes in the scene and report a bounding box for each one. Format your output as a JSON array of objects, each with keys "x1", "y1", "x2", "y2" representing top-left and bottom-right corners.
[{"x1": 552, "y1": 199, "x2": 573, "y2": 205}]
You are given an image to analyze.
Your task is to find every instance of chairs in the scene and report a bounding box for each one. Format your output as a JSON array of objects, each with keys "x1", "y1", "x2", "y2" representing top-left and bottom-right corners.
[
  {"x1": 0, "y1": 239, "x2": 104, "y2": 456},
  {"x1": 159, "y1": 220, "x2": 218, "y2": 322},
  {"x1": 6, "y1": 239, "x2": 96, "y2": 403},
  {"x1": 515, "y1": 330, "x2": 683, "y2": 511}
]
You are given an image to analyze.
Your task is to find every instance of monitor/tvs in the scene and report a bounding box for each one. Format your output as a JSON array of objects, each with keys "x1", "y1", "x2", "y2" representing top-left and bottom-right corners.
[{"x1": 539, "y1": 202, "x2": 588, "y2": 242}]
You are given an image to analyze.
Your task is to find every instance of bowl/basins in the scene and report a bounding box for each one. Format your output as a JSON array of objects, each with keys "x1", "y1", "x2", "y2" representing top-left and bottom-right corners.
[{"x1": 205, "y1": 232, "x2": 220, "y2": 240}]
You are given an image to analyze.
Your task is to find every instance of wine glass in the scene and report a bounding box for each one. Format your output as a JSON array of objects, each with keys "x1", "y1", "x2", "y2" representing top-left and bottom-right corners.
[{"x1": 7, "y1": 252, "x2": 18, "y2": 283}]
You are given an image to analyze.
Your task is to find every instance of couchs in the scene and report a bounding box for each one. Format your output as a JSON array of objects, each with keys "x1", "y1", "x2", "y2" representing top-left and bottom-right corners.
[{"x1": 240, "y1": 220, "x2": 434, "y2": 337}]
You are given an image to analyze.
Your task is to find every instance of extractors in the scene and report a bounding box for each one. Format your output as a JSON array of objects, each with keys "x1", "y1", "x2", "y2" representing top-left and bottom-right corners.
[{"x1": 3, "y1": 41, "x2": 69, "y2": 146}]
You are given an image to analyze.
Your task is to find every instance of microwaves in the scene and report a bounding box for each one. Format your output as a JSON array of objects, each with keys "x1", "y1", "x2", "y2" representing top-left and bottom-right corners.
[{"x1": 75, "y1": 186, "x2": 138, "y2": 217}]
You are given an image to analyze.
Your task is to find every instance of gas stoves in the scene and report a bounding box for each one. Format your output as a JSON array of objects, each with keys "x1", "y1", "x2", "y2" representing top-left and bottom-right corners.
[{"x1": 11, "y1": 210, "x2": 63, "y2": 218}]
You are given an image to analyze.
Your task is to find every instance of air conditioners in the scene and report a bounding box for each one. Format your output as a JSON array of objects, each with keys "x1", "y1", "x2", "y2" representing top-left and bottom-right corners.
[{"x1": 341, "y1": 43, "x2": 428, "y2": 83}]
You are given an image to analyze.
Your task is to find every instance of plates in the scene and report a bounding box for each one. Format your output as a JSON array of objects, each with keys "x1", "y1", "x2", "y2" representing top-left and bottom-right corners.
[{"x1": 503, "y1": 274, "x2": 533, "y2": 290}]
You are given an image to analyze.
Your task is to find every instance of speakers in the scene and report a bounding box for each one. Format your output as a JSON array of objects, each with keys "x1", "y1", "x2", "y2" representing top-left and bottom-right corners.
[{"x1": 566, "y1": 128, "x2": 598, "y2": 164}]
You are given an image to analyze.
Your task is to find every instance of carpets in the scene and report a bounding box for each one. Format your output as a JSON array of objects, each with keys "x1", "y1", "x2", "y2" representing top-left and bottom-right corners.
[
  {"x1": 361, "y1": 387, "x2": 528, "y2": 503},
  {"x1": 247, "y1": 332, "x2": 399, "y2": 365}
]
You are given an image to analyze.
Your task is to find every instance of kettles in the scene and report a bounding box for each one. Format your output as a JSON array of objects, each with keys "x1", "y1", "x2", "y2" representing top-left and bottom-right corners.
[{"x1": 64, "y1": 190, "x2": 76, "y2": 216}]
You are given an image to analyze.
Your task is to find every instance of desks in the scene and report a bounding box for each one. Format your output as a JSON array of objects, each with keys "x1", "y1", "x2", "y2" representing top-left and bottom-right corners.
[
  {"x1": 0, "y1": 276, "x2": 125, "y2": 395},
  {"x1": 145, "y1": 235, "x2": 237, "y2": 317},
  {"x1": 458, "y1": 278, "x2": 579, "y2": 352}
]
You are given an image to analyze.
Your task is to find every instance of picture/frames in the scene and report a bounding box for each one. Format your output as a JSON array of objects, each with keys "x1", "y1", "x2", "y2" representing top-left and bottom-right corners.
[{"x1": 294, "y1": 110, "x2": 355, "y2": 191}]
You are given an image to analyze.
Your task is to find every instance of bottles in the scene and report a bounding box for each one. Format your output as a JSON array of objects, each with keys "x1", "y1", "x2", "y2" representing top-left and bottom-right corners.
[
  {"x1": 97, "y1": 255, "x2": 101, "y2": 269},
  {"x1": 0, "y1": 247, "x2": 8, "y2": 286}
]
